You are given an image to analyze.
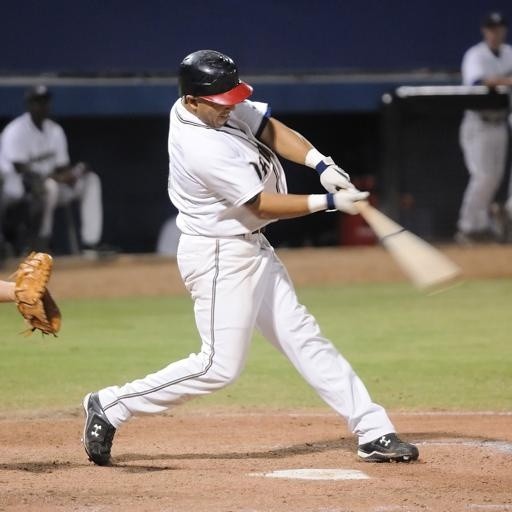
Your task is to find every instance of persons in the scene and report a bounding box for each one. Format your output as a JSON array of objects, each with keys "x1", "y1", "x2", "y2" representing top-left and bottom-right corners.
[
  {"x1": 153, "y1": 167, "x2": 428, "y2": 256},
  {"x1": 1, "y1": 84, "x2": 103, "y2": 262},
  {"x1": 0, "y1": 251, "x2": 60, "y2": 338},
  {"x1": 80, "y1": 47, "x2": 420, "y2": 466},
  {"x1": 451, "y1": 7, "x2": 512, "y2": 246}
]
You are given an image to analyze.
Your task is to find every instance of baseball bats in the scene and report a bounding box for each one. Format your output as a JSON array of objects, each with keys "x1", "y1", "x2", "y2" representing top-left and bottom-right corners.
[{"x1": 340, "y1": 188, "x2": 463, "y2": 297}]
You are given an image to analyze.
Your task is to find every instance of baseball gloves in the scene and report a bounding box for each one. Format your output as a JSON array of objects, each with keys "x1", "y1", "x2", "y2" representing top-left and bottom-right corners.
[{"x1": 13, "y1": 252, "x2": 61, "y2": 335}]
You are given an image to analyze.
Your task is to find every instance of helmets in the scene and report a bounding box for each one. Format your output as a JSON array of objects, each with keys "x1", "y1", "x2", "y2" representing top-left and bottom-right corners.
[{"x1": 173, "y1": 46, "x2": 256, "y2": 107}]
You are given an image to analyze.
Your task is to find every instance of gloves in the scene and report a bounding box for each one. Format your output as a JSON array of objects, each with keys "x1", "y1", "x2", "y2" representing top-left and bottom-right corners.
[
  {"x1": 308, "y1": 189, "x2": 370, "y2": 217},
  {"x1": 305, "y1": 147, "x2": 358, "y2": 192}
]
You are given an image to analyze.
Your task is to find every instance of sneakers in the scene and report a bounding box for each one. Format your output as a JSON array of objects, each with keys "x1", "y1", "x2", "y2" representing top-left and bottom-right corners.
[
  {"x1": 78, "y1": 390, "x2": 116, "y2": 465},
  {"x1": 357, "y1": 433, "x2": 421, "y2": 463}
]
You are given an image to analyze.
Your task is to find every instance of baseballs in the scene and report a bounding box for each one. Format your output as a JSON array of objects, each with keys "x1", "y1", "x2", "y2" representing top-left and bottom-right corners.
[{"x1": 382, "y1": 93, "x2": 393, "y2": 105}]
